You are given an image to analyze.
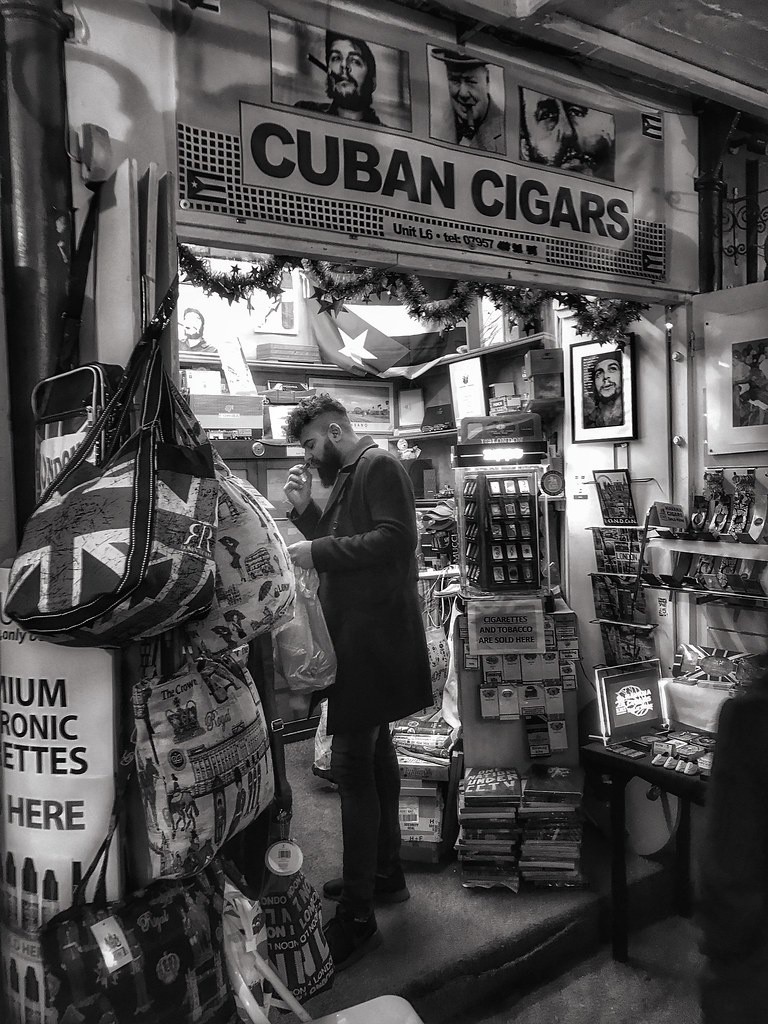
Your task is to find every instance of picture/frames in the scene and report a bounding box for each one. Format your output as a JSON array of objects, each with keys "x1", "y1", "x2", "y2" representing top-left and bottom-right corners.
[
  {"x1": 568, "y1": 332, "x2": 638, "y2": 443},
  {"x1": 307, "y1": 375, "x2": 398, "y2": 435}
]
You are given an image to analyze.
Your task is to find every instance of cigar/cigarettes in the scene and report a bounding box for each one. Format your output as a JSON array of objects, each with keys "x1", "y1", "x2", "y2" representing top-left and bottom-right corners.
[{"x1": 301, "y1": 463, "x2": 310, "y2": 472}]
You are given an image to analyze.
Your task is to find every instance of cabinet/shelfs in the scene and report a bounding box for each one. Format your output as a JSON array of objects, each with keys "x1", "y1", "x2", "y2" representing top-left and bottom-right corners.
[{"x1": 584, "y1": 457, "x2": 768, "y2": 960}]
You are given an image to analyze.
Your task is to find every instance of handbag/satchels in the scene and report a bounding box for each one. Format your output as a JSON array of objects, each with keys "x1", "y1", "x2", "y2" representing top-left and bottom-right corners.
[
  {"x1": 275, "y1": 558, "x2": 337, "y2": 696},
  {"x1": 131, "y1": 629, "x2": 275, "y2": 879},
  {"x1": 414, "y1": 623, "x2": 450, "y2": 717},
  {"x1": 165, "y1": 370, "x2": 295, "y2": 653},
  {"x1": 40, "y1": 772, "x2": 335, "y2": 1024},
  {"x1": 311, "y1": 698, "x2": 339, "y2": 783},
  {"x1": 5, "y1": 312, "x2": 219, "y2": 646}
]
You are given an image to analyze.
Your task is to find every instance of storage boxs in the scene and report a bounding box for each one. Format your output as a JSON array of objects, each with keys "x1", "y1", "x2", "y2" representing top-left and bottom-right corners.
[
  {"x1": 528, "y1": 373, "x2": 561, "y2": 398},
  {"x1": 523, "y1": 348, "x2": 564, "y2": 381}
]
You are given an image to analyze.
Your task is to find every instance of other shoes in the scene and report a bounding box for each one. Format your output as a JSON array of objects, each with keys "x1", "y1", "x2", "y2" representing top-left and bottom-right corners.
[
  {"x1": 323, "y1": 878, "x2": 410, "y2": 906},
  {"x1": 322, "y1": 904, "x2": 377, "y2": 964}
]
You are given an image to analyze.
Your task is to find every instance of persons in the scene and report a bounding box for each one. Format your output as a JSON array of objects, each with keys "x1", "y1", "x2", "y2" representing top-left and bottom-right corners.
[
  {"x1": 732, "y1": 337, "x2": 768, "y2": 427},
  {"x1": 284, "y1": 392, "x2": 435, "y2": 971},
  {"x1": 519, "y1": 87, "x2": 607, "y2": 179},
  {"x1": 582, "y1": 352, "x2": 625, "y2": 430},
  {"x1": 429, "y1": 47, "x2": 507, "y2": 156},
  {"x1": 177, "y1": 308, "x2": 218, "y2": 354},
  {"x1": 293, "y1": 30, "x2": 387, "y2": 127}
]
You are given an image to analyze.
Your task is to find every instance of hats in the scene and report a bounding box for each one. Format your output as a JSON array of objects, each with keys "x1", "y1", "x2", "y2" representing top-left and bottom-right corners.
[{"x1": 430, "y1": 47, "x2": 489, "y2": 65}]
